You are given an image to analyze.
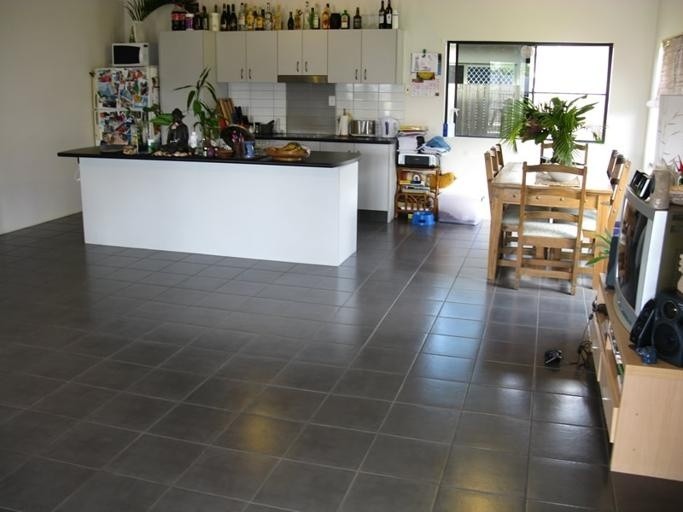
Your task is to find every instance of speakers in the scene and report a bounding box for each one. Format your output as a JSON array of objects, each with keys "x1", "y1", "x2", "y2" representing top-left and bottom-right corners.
[
  {"x1": 628, "y1": 298, "x2": 656, "y2": 351},
  {"x1": 650, "y1": 288, "x2": 683, "y2": 368}
]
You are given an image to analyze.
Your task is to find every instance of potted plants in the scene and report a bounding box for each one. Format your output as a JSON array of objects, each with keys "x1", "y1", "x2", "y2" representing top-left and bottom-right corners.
[
  {"x1": 120, "y1": 0, "x2": 147, "y2": 43},
  {"x1": 499, "y1": 90, "x2": 599, "y2": 182}
]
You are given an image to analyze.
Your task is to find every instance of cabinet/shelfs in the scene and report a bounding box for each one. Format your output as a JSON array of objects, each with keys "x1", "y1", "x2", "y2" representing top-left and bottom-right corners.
[
  {"x1": 215, "y1": 30, "x2": 278, "y2": 85},
  {"x1": 278, "y1": 30, "x2": 328, "y2": 76},
  {"x1": 320, "y1": 140, "x2": 388, "y2": 212},
  {"x1": 328, "y1": 28, "x2": 404, "y2": 86}
]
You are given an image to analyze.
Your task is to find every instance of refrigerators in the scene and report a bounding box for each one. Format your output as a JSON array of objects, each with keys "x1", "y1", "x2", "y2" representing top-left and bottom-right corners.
[{"x1": 90, "y1": 67, "x2": 160, "y2": 146}]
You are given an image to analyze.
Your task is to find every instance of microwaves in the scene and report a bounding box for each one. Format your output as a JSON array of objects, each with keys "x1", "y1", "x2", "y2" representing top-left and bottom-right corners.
[{"x1": 111, "y1": 43, "x2": 158, "y2": 67}]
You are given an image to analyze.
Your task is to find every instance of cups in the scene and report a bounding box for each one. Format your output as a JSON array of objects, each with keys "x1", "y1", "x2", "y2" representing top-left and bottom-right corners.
[{"x1": 243, "y1": 140, "x2": 255, "y2": 158}]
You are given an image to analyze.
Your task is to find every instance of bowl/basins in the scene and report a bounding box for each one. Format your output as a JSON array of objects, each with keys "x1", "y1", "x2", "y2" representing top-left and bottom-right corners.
[
  {"x1": 258, "y1": 124, "x2": 274, "y2": 137},
  {"x1": 416, "y1": 71, "x2": 435, "y2": 79},
  {"x1": 410, "y1": 211, "x2": 435, "y2": 225},
  {"x1": 670, "y1": 191, "x2": 683, "y2": 207},
  {"x1": 218, "y1": 150, "x2": 234, "y2": 157},
  {"x1": 351, "y1": 120, "x2": 375, "y2": 137}
]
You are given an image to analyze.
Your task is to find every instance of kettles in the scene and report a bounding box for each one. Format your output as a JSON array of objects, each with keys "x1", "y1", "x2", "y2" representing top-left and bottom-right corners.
[{"x1": 377, "y1": 115, "x2": 399, "y2": 138}]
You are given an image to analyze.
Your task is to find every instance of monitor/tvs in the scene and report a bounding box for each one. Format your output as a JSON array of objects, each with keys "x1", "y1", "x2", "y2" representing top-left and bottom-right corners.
[{"x1": 612, "y1": 183, "x2": 683, "y2": 339}]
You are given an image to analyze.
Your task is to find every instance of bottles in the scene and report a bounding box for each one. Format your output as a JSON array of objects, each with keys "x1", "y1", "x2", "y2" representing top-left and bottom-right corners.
[
  {"x1": 339, "y1": 108, "x2": 349, "y2": 137},
  {"x1": 190, "y1": 131, "x2": 198, "y2": 148},
  {"x1": 202, "y1": 146, "x2": 218, "y2": 159},
  {"x1": 254, "y1": 122, "x2": 260, "y2": 134},
  {"x1": 248, "y1": 123, "x2": 253, "y2": 136},
  {"x1": 378, "y1": 0, "x2": 399, "y2": 29},
  {"x1": 129, "y1": 25, "x2": 134, "y2": 42},
  {"x1": 146, "y1": 122, "x2": 157, "y2": 154},
  {"x1": 171, "y1": 1, "x2": 363, "y2": 31}
]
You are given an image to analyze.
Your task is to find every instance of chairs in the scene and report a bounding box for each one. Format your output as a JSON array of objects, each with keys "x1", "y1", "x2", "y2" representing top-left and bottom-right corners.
[{"x1": 483, "y1": 139, "x2": 633, "y2": 296}]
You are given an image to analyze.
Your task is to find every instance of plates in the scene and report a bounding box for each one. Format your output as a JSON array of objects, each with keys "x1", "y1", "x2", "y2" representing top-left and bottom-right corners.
[
  {"x1": 98, "y1": 147, "x2": 122, "y2": 153},
  {"x1": 264, "y1": 147, "x2": 312, "y2": 162}
]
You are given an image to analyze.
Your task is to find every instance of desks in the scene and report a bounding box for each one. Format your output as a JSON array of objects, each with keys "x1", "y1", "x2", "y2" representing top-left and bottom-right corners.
[{"x1": 587, "y1": 271, "x2": 683, "y2": 491}]
